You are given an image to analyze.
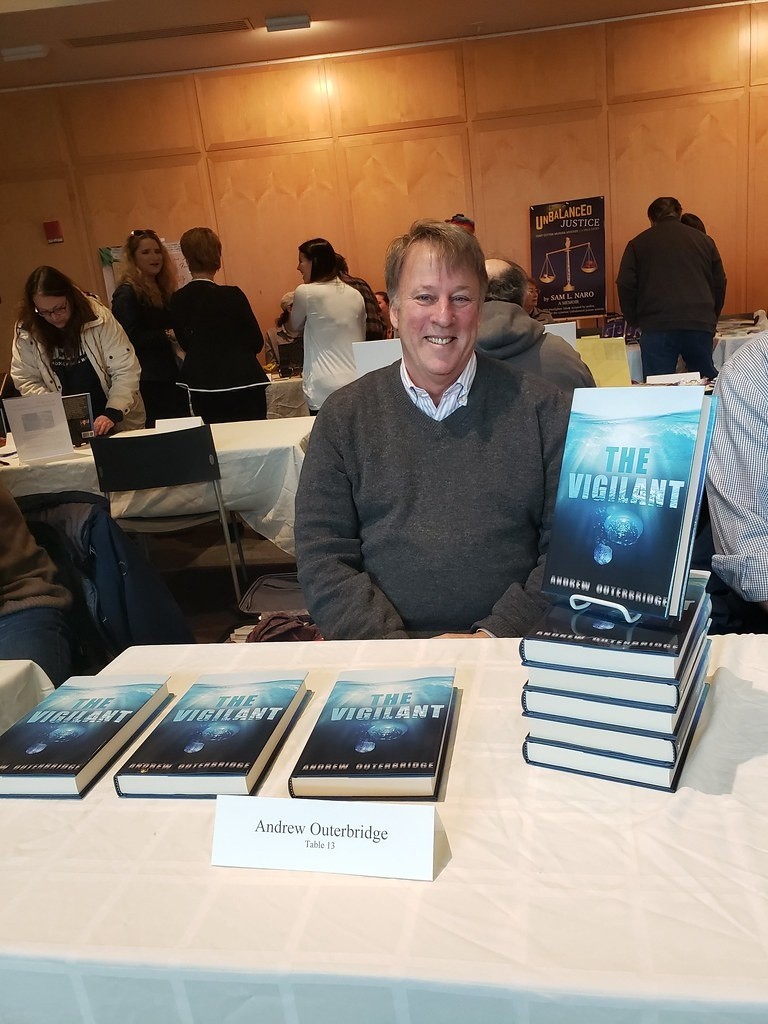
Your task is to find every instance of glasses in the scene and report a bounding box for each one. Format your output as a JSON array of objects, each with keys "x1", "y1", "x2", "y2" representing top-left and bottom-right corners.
[
  {"x1": 34, "y1": 297, "x2": 69, "y2": 316},
  {"x1": 127, "y1": 229, "x2": 158, "y2": 245}
]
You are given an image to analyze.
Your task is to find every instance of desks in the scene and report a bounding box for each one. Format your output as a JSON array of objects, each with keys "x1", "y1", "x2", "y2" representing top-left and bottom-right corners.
[
  {"x1": 0, "y1": 436, "x2": 103, "y2": 498},
  {"x1": 106, "y1": 415, "x2": 317, "y2": 558},
  {"x1": 625, "y1": 343, "x2": 643, "y2": 378},
  {"x1": 714, "y1": 319, "x2": 762, "y2": 370},
  {"x1": 0, "y1": 630, "x2": 768, "y2": 1024},
  {"x1": 265, "y1": 374, "x2": 309, "y2": 418}
]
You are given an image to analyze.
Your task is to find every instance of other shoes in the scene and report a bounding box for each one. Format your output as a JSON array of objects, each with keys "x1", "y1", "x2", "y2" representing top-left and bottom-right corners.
[{"x1": 224, "y1": 521, "x2": 242, "y2": 542}]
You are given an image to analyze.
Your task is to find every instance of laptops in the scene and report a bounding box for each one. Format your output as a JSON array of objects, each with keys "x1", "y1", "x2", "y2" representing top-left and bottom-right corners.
[{"x1": 60, "y1": 393, "x2": 97, "y2": 447}]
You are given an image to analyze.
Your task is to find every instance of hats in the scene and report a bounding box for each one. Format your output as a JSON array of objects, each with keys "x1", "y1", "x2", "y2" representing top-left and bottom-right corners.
[{"x1": 281, "y1": 291, "x2": 298, "y2": 312}]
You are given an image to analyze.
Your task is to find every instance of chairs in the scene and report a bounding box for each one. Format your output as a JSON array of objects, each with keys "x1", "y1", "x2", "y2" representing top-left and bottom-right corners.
[
  {"x1": 576, "y1": 336, "x2": 632, "y2": 387},
  {"x1": 10, "y1": 486, "x2": 133, "y2": 668},
  {"x1": 85, "y1": 422, "x2": 249, "y2": 610}
]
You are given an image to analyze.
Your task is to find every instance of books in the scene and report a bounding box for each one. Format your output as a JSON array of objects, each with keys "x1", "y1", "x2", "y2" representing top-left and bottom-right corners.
[
  {"x1": 287, "y1": 666, "x2": 459, "y2": 803},
  {"x1": 113, "y1": 671, "x2": 312, "y2": 799},
  {"x1": 517, "y1": 569, "x2": 712, "y2": 794},
  {"x1": 542, "y1": 385, "x2": 720, "y2": 622},
  {"x1": 0, "y1": 674, "x2": 174, "y2": 799}
]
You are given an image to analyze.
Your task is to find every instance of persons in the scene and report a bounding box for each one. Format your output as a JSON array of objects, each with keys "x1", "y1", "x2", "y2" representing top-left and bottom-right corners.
[
  {"x1": 445, "y1": 213, "x2": 596, "y2": 398},
  {"x1": 111, "y1": 229, "x2": 193, "y2": 429},
  {"x1": 615, "y1": 196, "x2": 727, "y2": 383},
  {"x1": 294, "y1": 218, "x2": 570, "y2": 640},
  {"x1": 0, "y1": 485, "x2": 73, "y2": 689},
  {"x1": 704, "y1": 327, "x2": 768, "y2": 612},
  {"x1": 264, "y1": 253, "x2": 400, "y2": 374},
  {"x1": 288, "y1": 237, "x2": 367, "y2": 416},
  {"x1": 10, "y1": 266, "x2": 141, "y2": 436},
  {"x1": 170, "y1": 227, "x2": 271, "y2": 423}
]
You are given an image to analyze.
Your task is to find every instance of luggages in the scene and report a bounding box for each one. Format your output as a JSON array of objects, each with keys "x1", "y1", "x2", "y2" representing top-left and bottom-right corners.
[{"x1": 214, "y1": 570, "x2": 312, "y2": 643}]
